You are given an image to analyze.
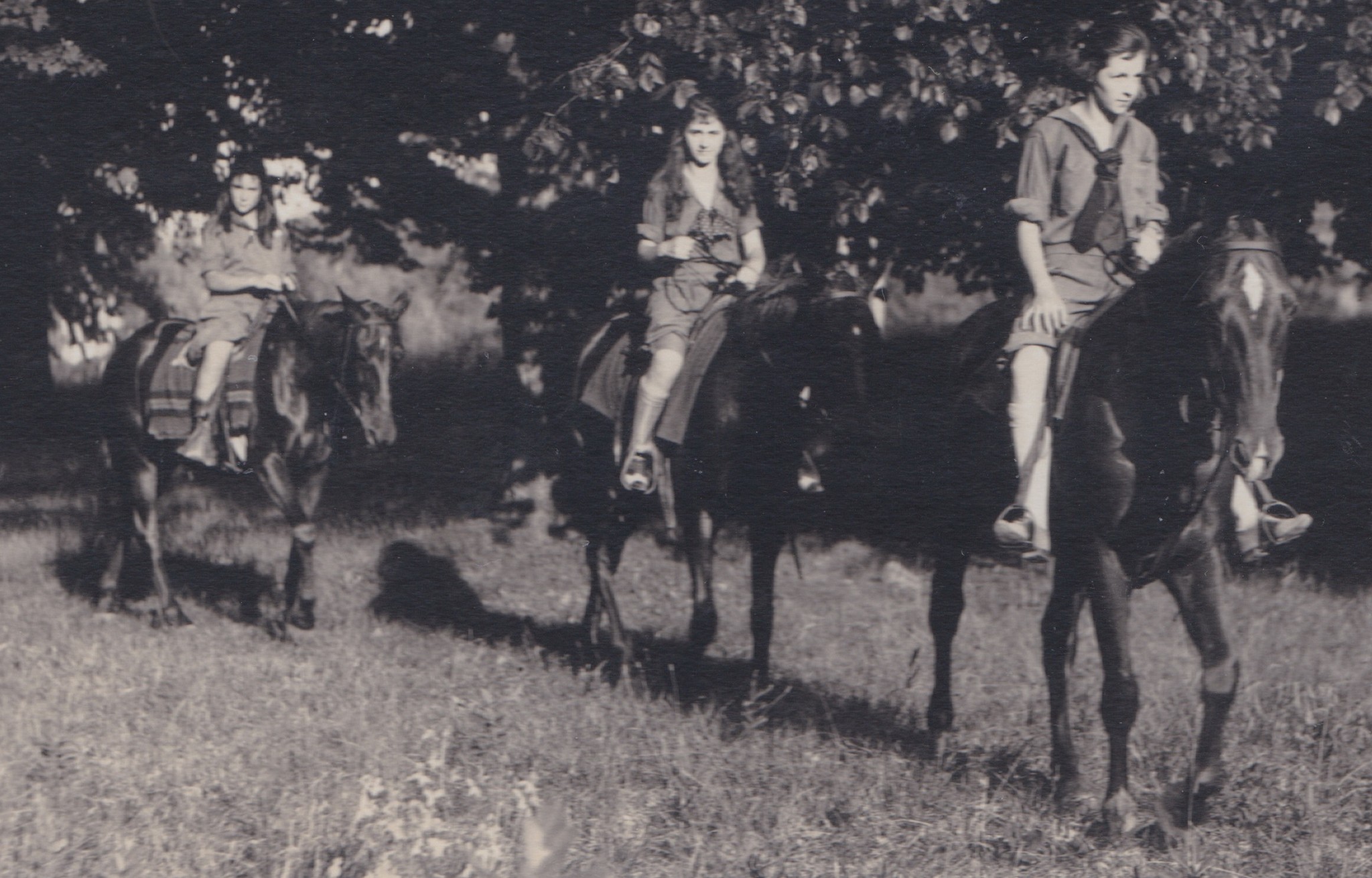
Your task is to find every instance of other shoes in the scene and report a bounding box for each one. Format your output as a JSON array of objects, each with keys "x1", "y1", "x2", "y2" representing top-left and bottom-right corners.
[
  {"x1": 1233, "y1": 510, "x2": 1313, "y2": 564},
  {"x1": 994, "y1": 514, "x2": 1049, "y2": 566}
]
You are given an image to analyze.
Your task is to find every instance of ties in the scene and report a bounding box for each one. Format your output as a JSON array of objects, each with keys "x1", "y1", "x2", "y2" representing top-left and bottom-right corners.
[{"x1": 1069, "y1": 121, "x2": 1129, "y2": 255}]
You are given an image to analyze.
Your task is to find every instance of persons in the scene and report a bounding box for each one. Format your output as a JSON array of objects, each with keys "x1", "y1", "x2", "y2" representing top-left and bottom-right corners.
[
  {"x1": 989, "y1": 20, "x2": 1314, "y2": 569},
  {"x1": 175, "y1": 155, "x2": 299, "y2": 468},
  {"x1": 619, "y1": 98, "x2": 768, "y2": 492}
]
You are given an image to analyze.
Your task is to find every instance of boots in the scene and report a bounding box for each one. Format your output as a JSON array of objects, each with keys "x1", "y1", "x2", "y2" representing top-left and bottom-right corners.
[
  {"x1": 796, "y1": 461, "x2": 824, "y2": 491},
  {"x1": 175, "y1": 399, "x2": 220, "y2": 468},
  {"x1": 620, "y1": 377, "x2": 672, "y2": 493}
]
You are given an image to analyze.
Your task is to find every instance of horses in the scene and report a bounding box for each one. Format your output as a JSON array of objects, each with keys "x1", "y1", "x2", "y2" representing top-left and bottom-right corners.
[
  {"x1": 924, "y1": 207, "x2": 1298, "y2": 844},
  {"x1": 99, "y1": 284, "x2": 411, "y2": 644},
  {"x1": 566, "y1": 271, "x2": 886, "y2": 711}
]
